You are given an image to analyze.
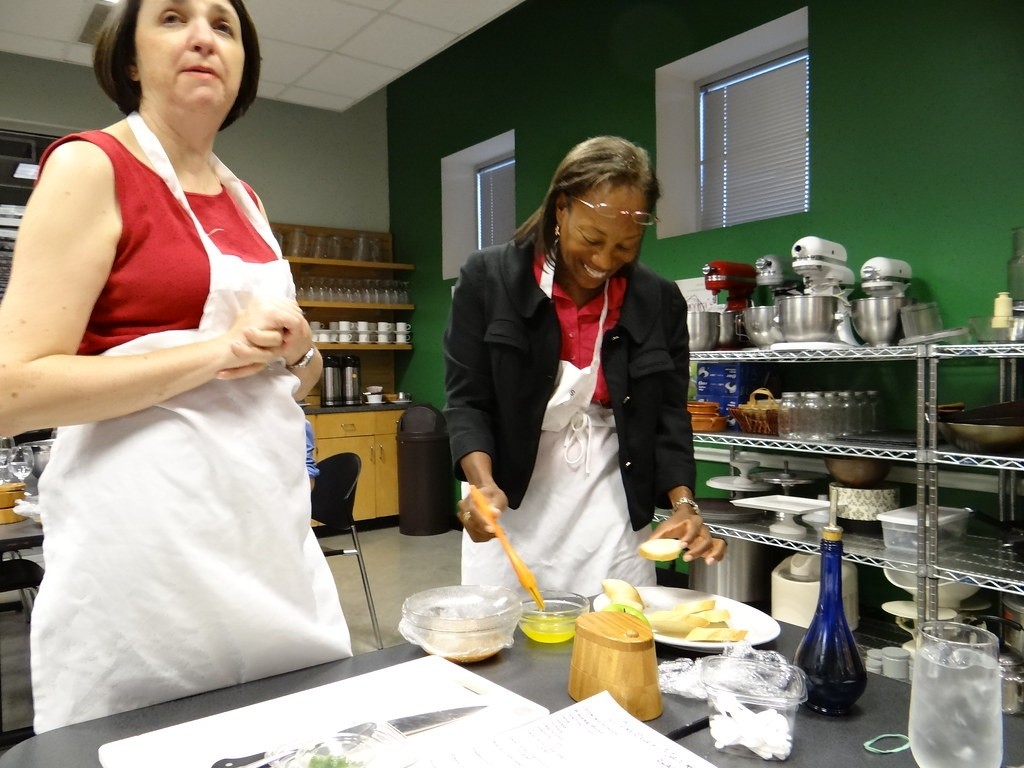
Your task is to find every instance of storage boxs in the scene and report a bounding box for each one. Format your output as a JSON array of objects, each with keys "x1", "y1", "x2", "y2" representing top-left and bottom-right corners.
[{"x1": 877, "y1": 503, "x2": 971, "y2": 554}]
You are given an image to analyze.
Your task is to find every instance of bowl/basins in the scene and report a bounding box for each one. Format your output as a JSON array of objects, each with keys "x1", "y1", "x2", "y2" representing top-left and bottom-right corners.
[
  {"x1": 20, "y1": 439, "x2": 55, "y2": 478},
  {"x1": 826, "y1": 454, "x2": 889, "y2": 489},
  {"x1": 882, "y1": 557, "x2": 981, "y2": 608},
  {"x1": 401, "y1": 584, "x2": 523, "y2": 664},
  {"x1": 687, "y1": 294, "x2": 1024, "y2": 353},
  {"x1": 936, "y1": 399, "x2": 1024, "y2": 453},
  {"x1": 519, "y1": 590, "x2": 590, "y2": 643}
]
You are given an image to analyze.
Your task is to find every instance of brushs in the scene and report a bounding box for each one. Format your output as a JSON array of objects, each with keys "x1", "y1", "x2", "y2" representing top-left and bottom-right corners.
[{"x1": 469, "y1": 484, "x2": 546, "y2": 610}]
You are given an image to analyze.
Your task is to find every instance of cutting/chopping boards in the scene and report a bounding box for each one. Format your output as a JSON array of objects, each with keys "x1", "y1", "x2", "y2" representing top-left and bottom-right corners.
[{"x1": 98, "y1": 655, "x2": 551, "y2": 768}]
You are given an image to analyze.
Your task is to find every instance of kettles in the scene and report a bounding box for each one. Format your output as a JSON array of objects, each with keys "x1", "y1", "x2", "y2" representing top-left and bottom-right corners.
[{"x1": 957, "y1": 614, "x2": 1024, "y2": 718}]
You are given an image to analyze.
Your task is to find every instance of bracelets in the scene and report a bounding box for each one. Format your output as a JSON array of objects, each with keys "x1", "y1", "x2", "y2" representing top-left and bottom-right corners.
[
  {"x1": 673, "y1": 497, "x2": 701, "y2": 515},
  {"x1": 287, "y1": 346, "x2": 315, "y2": 369}
]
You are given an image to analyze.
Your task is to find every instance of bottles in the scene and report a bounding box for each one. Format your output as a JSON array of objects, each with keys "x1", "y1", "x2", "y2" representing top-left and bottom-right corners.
[
  {"x1": 777, "y1": 388, "x2": 882, "y2": 441},
  {"x1": 794, "y1": 526, "x2": 868, "y2": 716},
  {"x1": 342, "y1": 354, "x2": 361, "y2": 407},
  {"x1": 322, "y1": 355, "x2": 342, "y2": 407}
]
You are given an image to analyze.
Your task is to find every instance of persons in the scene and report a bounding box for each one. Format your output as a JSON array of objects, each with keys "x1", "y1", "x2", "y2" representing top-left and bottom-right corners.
[
  {"x1": 442, "y1": 136, "x2": 726, "y2": 604},
  {"x1": 0, "y1": 1, "x2": 354, "y2": 735},
  {"x1": 305, "y1": 418, "x2": 320, "y2": 492}
]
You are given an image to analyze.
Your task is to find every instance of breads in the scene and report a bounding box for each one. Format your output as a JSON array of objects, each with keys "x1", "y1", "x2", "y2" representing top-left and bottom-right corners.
[
  {"x1": 601, "y1": 578, "x2": 748, "y2": 641},
  {"x1": 637, "y1": 538, "x2": 682, "y2": 561}
]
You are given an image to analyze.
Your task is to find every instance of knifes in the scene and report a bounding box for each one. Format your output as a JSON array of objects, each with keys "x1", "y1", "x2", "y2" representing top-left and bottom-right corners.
[{"x1": 207, "y1": 705, "x2": 487, "y2": 768}]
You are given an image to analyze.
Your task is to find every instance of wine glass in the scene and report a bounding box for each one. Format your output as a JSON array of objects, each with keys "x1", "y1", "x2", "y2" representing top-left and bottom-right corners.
[
  {"x1": 0, "y1": 436, "x2": 35, "y2": 496},
  {"x1": 292, "y1": 277, "x2": 409, "y2": 303}
]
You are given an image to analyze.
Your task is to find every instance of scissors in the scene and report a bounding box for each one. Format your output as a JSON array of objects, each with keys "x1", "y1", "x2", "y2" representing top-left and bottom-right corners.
[{"x1": 211, "y1": 705, "x2": 489, "y2": 768}]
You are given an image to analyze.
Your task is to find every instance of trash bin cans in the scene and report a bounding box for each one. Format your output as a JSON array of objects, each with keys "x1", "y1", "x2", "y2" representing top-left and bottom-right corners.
[{"x1": 395, "y1": 405, "x2": 452, "y2": 536}]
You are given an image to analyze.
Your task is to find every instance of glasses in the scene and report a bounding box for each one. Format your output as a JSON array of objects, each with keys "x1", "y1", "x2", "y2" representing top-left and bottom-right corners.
[{"x1": 571, "y1": 196, "x2": 661, "y2": 226}]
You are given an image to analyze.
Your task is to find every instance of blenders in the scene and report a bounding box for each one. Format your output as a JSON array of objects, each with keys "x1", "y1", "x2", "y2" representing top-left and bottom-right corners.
[{"x1": 702, "y1": 235, "x2": 911, "y2": 351}]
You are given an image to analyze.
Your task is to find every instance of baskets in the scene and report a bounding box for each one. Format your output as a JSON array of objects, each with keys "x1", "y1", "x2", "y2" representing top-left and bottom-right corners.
[{"x1": 730, "y1": 388, "x2": 794, "y2": 437}]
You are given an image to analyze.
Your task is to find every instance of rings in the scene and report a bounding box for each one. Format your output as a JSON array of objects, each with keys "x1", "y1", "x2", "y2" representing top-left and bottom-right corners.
[{"x1": 463, "y1": 511, "x2": 472, "y2": 521}]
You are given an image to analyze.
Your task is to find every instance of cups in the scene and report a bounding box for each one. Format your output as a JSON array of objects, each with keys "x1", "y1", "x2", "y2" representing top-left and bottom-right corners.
[
  {"x1": 311, "y1": 319, "x2": 411, "y2": 344},
  {"x1": 700, "y1": 656, "x2": 808, "y2": 758},
  {"x1": 271, "y1": 229, "x2": 384, "y2": 261},
  {"x1": 367, "y1": 394, "x2": 383, "y2": 403},
  {"x1": 909, "y1": 620, "x2": 1004, "y2": 768}
]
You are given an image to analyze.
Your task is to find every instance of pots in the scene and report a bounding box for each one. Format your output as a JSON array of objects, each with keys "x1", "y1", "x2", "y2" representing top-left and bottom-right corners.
[{"x1": 687, "y1": 533, "x2": 787, "y2": 604}]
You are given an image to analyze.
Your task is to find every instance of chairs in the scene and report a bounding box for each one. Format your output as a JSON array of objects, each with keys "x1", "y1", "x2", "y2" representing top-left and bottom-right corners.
[
  {"x1": 309, "y1": 451, "x2": 385, "y2": 651},
  {"x1": 1, "y1": 548, "x2": 45, "y2": 624}
]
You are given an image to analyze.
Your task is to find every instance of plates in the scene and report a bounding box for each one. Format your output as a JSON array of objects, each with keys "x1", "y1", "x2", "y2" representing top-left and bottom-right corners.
[
  {"x1": 366, "y1": 401, "x2": 387, "y2": 405},
  {"x1": 391, "y1": 400, "x2": 412, "y2": 403},
  {"x1": 592, "y1": 585, "x2": 780, "y2": 653}
]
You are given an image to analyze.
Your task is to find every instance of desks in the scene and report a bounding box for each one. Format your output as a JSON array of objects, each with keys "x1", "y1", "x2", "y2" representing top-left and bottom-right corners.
[{"x1": 0, "y1": 592, "x2": 1024, "y2": 768}]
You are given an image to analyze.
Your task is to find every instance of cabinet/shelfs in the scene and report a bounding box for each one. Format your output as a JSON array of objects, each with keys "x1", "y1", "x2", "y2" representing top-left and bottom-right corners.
[
  {"x1": 652, "y1": 346, "x2": 1024, "y2": 661},
  {"x1": 268, "y1": 218, "x2": 415, "y2": 528}
]
